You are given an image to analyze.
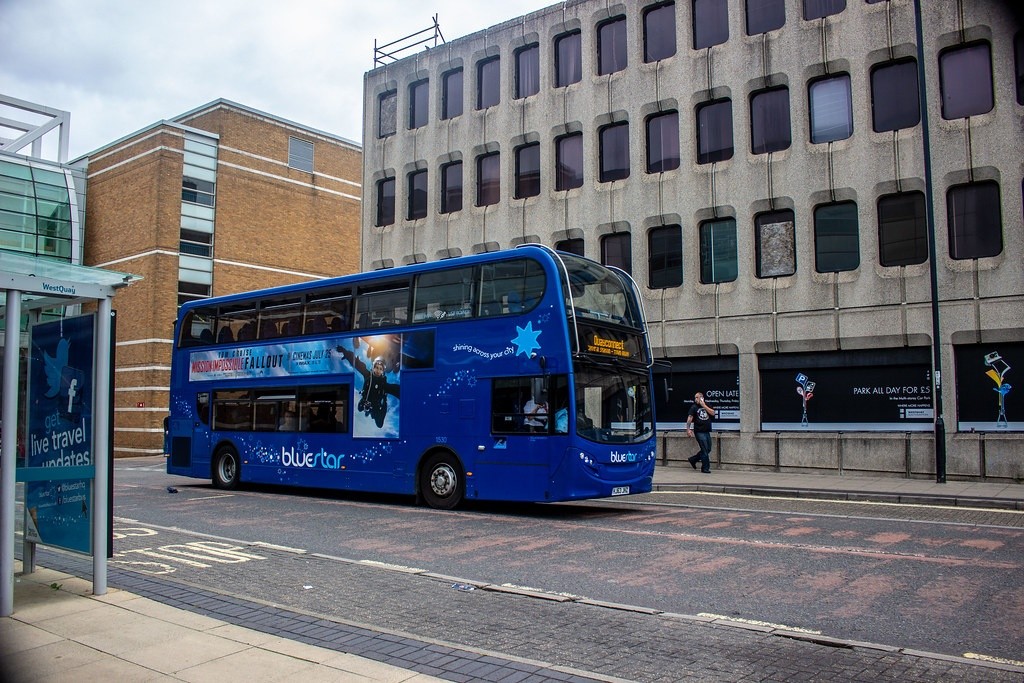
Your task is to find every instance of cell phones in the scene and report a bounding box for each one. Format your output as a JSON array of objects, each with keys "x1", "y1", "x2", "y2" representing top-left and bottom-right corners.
[{"x1": 700, "y1": 397, "x2": 704, "y2": 402}]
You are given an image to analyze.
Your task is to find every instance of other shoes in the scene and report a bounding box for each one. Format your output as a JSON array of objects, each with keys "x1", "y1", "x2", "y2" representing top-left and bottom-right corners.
[
  {"x1": 688, "y1": 457, "x2": 697, "y2": 469},
  {"x1": 701, "y1": 469, "x2": 711, "y2": 473}
]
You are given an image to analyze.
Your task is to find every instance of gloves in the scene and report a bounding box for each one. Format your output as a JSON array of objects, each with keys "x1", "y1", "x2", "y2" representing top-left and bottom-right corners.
[{"x1": 336, "y1": 345, "x2": 344, "y2": 352}]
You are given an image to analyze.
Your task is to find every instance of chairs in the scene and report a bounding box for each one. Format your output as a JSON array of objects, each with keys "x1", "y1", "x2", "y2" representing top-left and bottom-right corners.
[{"x1": 199, "y1": 315, "x2": 345, "y2": 345}]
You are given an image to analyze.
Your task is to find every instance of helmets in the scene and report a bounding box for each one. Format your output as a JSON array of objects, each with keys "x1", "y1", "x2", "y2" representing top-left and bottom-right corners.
[{"x1": 373, "y1": 356, "x2": 387, "y2": 370}]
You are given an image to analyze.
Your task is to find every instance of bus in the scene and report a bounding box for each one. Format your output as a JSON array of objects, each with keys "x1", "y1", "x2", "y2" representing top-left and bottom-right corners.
[{"x1": 162, "y1": 243, "x2": 658, "y2": 510}]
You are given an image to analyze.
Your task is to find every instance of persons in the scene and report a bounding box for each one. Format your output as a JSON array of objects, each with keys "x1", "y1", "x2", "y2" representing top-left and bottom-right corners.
[
  {"x1": 336, "y1": 344, "x2": 387, "y2": 414},
  {"x1": 232, "y1": 396, "x2": 345, "y2": 432},
  {"x1": 523, "y1": 397, "x2": 548, "y2": 432},
  {"x1": 686, "y1": 392, "x2": 715, "y2": 473}
]
